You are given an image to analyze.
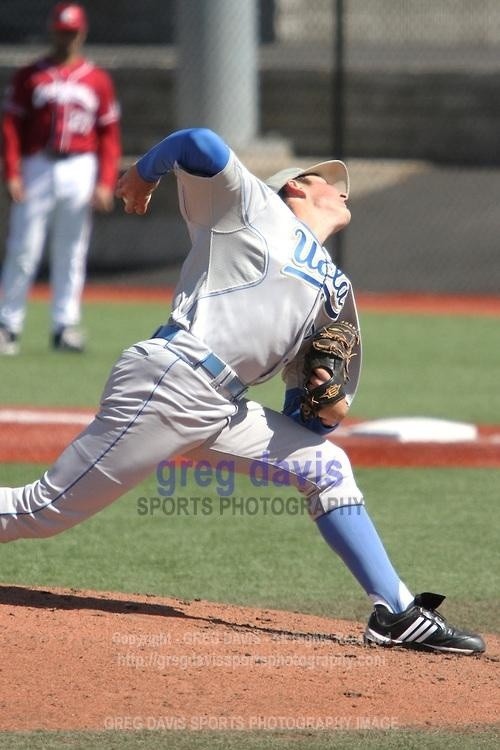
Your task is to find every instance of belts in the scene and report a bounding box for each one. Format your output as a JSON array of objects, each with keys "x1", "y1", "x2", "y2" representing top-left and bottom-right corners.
[{"x1": 153, "y1": 327, "x2": 249, "y2": 401}]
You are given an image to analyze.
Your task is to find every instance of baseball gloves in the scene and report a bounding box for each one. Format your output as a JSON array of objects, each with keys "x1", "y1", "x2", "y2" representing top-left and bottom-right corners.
[{"x1": 300, "y1": 321, "x2": 359, "y2": 424}]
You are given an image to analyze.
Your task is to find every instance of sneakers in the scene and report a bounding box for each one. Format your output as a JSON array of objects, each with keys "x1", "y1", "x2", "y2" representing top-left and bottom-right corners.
[
  {"x1": 0, "y1": 323, "x2": 20, "y2": 356},
  {"x1": 50, "y1": 326, "x2": 84, "y2": 352},
  {"x1": 363, "y1": 593, "x2": 485, "y2": 656}
]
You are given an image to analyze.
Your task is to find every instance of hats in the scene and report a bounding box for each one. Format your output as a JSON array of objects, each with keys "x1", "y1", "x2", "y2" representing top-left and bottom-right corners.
[
  {"x1": 51, "y1": 5, "x2": 84, "y2": 31},
  {"x1": 264, "y1": 160, "x2": 350, "y2": 195}
]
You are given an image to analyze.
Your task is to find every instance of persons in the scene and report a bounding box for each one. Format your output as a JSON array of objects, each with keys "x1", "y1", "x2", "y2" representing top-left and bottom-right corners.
[
  {"x1": 0, "y1": 3, "x2": 121, "y2": 356},
  {"x1": 0, "y1": 128, "x2": 486, "y2": 656}
]
